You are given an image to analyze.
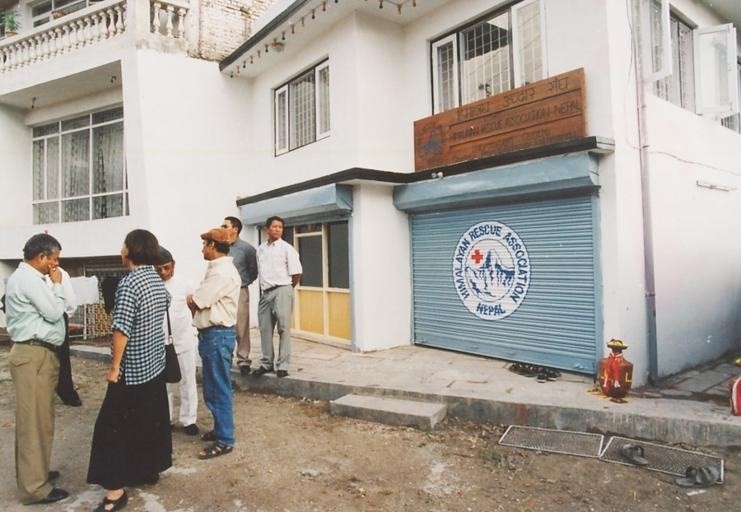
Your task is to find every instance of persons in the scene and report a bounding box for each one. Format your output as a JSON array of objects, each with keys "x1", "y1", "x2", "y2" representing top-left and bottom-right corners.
[
  {"x1": 4, "y1": 234, "x2": 70, "y2": 504},
  {"x1": 88, "y1": 228, "x2": 171, "y2": 511},
  {"x1": 219, "y1": 217, "x2": 256, "y2": 375},
  {"x1": 155, "y1": 247, "x2": 198, "y2": 433},
  {"x1": 252, "y1": 216, "x2": 303, "y2": 377},
  {"x1": 48, "y1": 267, "x2": 80, "y2": 405},
  {"x1": 188, "y1": 229, "x2": 244, "y2": 458}
]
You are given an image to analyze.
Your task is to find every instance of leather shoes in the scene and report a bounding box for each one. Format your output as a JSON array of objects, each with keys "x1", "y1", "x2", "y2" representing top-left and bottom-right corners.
[
  {"x1": 40, "y1": 488, "x2": 67, "y2": 502},
  {"x1": 277, "y1": 370, "x2": 287, "y2": 377},
  {"x1": 241, "y1": 366, "x2": 249, "y2": 376},
  {"x1": 253, "y1": 367, "x2": 271, "y2": 376},
  {"x1": 185, "y1": 424, "x2": 198, "y2": 435}
]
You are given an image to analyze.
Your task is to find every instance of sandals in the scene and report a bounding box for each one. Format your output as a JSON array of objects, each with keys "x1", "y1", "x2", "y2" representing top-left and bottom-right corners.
[
  {"x1": 201, "y1": 432, "x2": 216, "y2": 441},
  {"x1": 93, "y1": 489, "x2": 127, "y2": 512},
  {"x1": 199, "y1": 443, "x2": 232, "y2": 459}
]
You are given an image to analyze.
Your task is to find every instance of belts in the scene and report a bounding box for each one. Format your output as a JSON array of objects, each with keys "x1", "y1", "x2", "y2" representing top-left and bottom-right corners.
[{"x1": 16, "y1": 339, "x2": 62, "y2": 352}]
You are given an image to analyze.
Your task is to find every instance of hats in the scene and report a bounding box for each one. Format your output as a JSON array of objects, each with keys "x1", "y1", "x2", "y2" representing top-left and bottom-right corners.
[
  {"x1": 201, "y1": 228, "x2": 229, "y2": 245},
  {"x1": 157, "y1": 246, "x2": 172, "y2": 265}
]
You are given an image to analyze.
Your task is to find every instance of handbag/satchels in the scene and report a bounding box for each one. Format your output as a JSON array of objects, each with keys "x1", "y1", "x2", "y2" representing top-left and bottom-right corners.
[{"x1": 163, "y1": 345, "x2": 181, "y2": 383}]
